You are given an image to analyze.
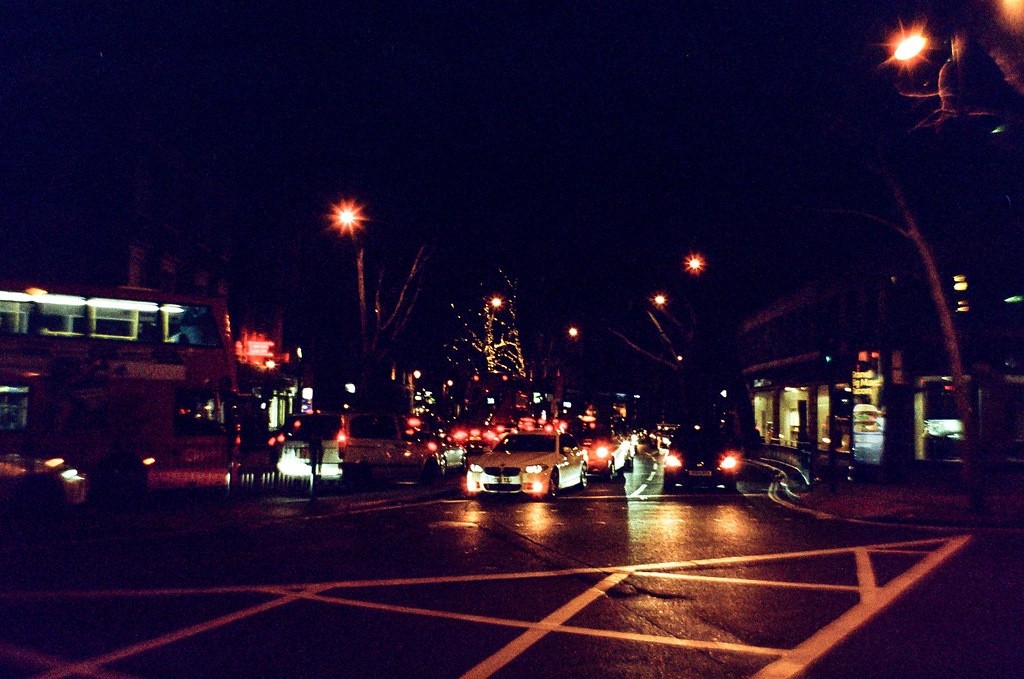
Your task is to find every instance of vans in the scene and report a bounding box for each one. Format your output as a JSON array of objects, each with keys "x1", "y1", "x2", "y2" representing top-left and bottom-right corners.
[{"x1": 277, "y1": 410, "x2": 447, "y2": 496}]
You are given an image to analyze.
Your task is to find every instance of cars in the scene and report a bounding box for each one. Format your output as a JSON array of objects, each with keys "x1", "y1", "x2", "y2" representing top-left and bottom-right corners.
[
  {"x1": 579, "y1": 423, "x2": 745, "y2": 491},
  {"x1": 465, "y1": 428, "x2": 591, "y2": 501}
]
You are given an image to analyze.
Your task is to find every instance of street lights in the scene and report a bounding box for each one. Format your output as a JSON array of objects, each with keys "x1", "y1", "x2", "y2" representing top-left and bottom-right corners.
[{"x1": 329, "y1": 195, "x2": 370, "y2": 365}]
